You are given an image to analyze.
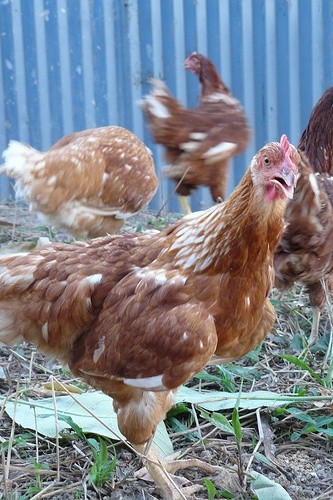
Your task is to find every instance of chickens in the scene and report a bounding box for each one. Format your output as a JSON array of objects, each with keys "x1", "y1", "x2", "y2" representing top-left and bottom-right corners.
[{"x1": 0, "y1": 52, "x2": 332, "y2": 499}]
